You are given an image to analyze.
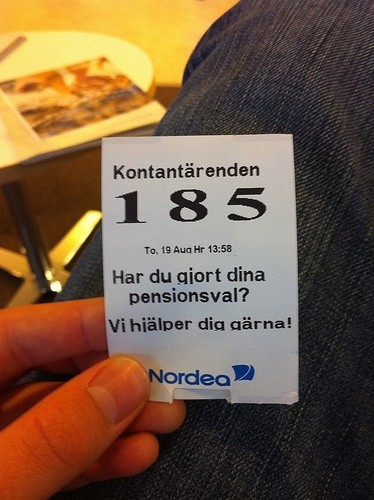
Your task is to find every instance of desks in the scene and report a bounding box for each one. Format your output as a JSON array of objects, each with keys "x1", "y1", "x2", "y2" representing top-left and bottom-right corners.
[{"x1": 0, "y1": 32, "x2": 156, "y2": 308}]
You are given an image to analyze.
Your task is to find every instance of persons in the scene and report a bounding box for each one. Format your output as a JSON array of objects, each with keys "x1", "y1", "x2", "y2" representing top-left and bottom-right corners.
[{"x1": 1, "y1": 2, "x2": 374, "y2": 499}]
